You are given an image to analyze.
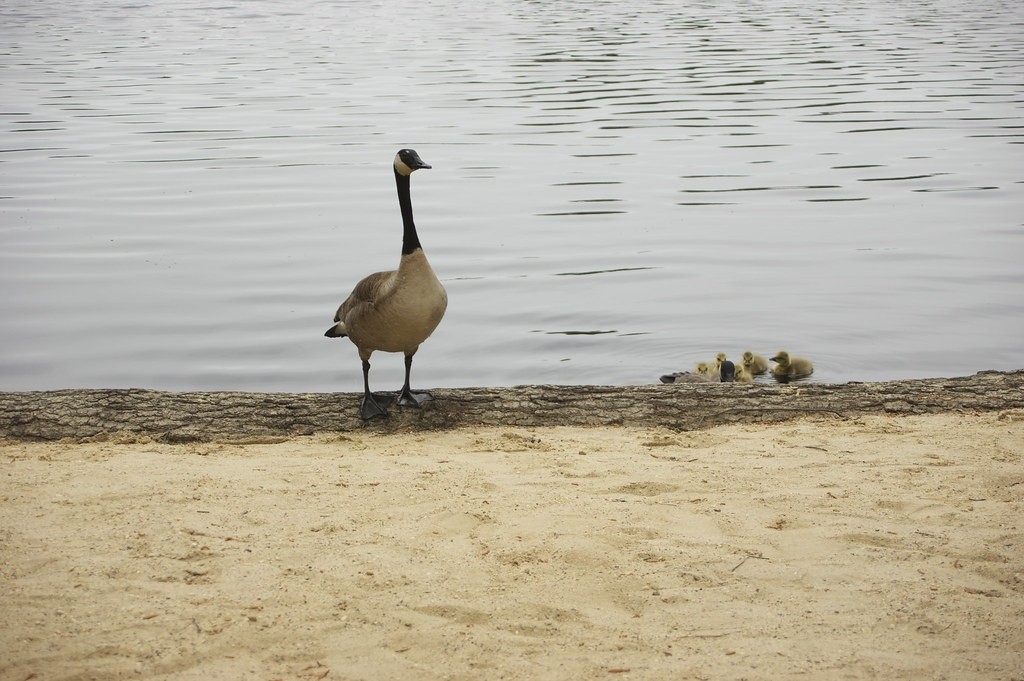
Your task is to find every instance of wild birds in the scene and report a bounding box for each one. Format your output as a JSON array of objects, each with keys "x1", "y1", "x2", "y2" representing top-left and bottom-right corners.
[
  {"x1": 657, "y1": 350, "x2": 812, "y2": 387},
  {"x1": 323, "y1": 147, "x2": 448, "y2": 421}
]
